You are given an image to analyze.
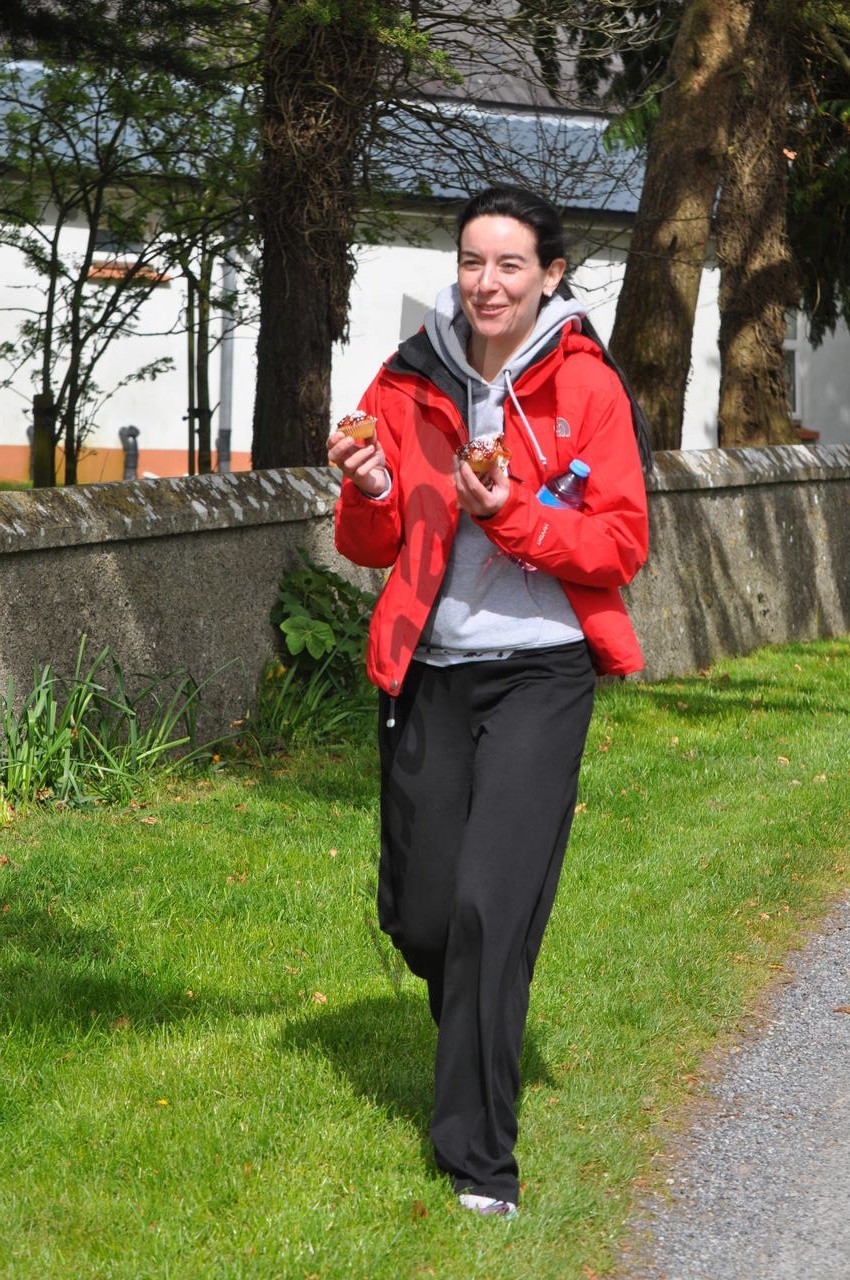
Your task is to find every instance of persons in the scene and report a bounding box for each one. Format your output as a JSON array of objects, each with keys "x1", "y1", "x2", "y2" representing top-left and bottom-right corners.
[{"x1": 326, "y1": 187, "x2": 648, "y2": 1226}]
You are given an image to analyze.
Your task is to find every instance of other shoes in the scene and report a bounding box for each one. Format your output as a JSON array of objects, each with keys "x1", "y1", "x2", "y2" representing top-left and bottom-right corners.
[{"x1": 458, "y1": 1194, "x2": 516, "y2": 1222}]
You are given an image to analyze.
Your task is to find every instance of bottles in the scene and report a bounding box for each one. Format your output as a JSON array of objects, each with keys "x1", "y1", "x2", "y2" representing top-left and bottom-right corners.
[{"x1": 499, "y1": 458, "x2": 590, "y2": 571}]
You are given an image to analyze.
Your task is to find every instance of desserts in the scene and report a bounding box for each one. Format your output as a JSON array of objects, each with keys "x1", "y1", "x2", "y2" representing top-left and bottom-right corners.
[
  {"x1": 335, "y1": 410, "x2": 377, "y2": 441},
  {"x1": 456, "y1": 439, "x2": 500, "y2": 473}
]
